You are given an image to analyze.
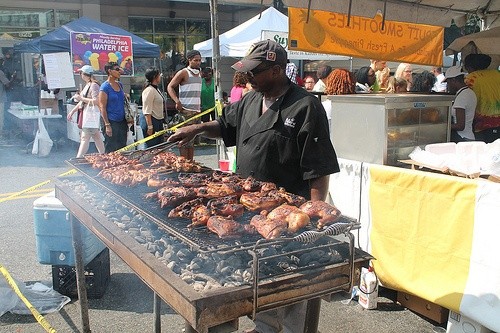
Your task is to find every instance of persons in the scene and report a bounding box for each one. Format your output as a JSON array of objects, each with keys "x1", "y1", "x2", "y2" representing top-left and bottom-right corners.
[
  {"x1": 140, "y1": 67, "x2": 165, "y2": 149},
  {"x1": 431, "y1": 66, "x2": 447, "y2": 92},
  {"x1": 464, "y1": 54, "x2": 500, "y2": 143},
  {"x1": 441, "y1": 66, "x2": 477, "y2": 144},
  {"x1": 167, "y1": 40, "x2": 340, "y2": 333},
  {"x1": 223, "y1": 92, "x2": 229, "y2": 107},
  {"x1": 322, "y1": 69, "x2": 355, "y2": 132},
  {"x1": 200, "y1": 68, "x2": 215, "y2": 145},
  {"x1": 395, "y1": 62, "x2": 413, "y2": 92},
  {"x1": 370, "y1": 60, "x2": 386, "y2": 92},
  {"x1": 98, "y1": 62, "x2": 129, "y2": 154},
  {"x1": 231, "y1": 71, "x2": 249, "y2": 103},
  {"x1": 303, "y1": 76, "x2": 316, "y2": 91},
  {"x1": 312, "y1": 65, "x2": 332, "y2": 102},
  {"x1": 409, "y1": 71, "x2": 437, "y2": 92},
  {"x1": 167, "y1": 50, "x2": 202, "y2": 160},
  {"x1": 67, "y1": 65, "x2": 105, "y2": 157},
  {"x1": 375, "y1": 67, "x2": 390, "y2": 92},
  {"x1": 354, "y1": 66, "x2": 376, "y2": 93},
  {"x1": 286, "y1": 63, "x2": 304, "y2": 87},
  {"x1": 387, "y1": 77, "x2": 407, "y2": 92},
  {"x1": 0, "y1": 59, "x2": 17, "y2": 140}
]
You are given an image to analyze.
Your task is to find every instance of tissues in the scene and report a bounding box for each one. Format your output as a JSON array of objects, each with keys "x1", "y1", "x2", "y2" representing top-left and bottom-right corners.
[{"x1": 226, "y1": 152, "x2": 236, "y2": 170}]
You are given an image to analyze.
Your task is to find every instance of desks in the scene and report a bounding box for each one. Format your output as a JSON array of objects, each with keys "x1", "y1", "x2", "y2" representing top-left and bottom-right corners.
[{"x1": 8, "y1": 108, "x2": 62, "y2": 154}]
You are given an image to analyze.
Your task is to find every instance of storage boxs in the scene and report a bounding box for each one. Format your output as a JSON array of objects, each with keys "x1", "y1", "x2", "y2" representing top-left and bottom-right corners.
[
  {"x1": 38, "y1": 97, "x2": 59, "y2": 114},
  {"x1": 51, "y1": 247, "x2": 111, "y2": 299},
  {"x1": 396, "y1": 291, "x2": 448, "y2": 323},
  {"x1": 33, "y1": 190, "x2": 106, "y2": 266},
  {"x1": 445, "y1": 309, "x2": 491, "y2": 332}
]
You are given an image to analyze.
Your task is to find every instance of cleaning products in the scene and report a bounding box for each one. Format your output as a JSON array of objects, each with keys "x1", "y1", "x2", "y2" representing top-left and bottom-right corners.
[{"x1": 357, "y1": 261, "x2": 379, "y2": 310}]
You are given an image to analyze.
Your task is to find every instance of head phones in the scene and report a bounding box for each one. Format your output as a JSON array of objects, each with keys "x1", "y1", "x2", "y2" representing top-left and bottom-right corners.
[{"x1": 187, "y1": 66, "x2": 201, "y2": 74}]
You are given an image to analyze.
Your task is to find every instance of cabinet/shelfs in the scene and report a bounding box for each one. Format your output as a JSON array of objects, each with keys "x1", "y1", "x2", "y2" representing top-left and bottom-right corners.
[{"x1": 327, "y1": 94, "x2": 456, "y2": 168}]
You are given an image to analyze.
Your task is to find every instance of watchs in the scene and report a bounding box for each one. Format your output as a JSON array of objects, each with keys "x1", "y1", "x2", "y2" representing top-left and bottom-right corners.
[{"x1": 148, "y1": 126, "x2": 153, "y2": 129}]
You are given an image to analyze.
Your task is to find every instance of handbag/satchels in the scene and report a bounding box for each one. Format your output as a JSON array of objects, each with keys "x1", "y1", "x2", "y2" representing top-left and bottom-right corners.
[
  {"x1": 78, "y1": 108, "x2": 82, "y2": 130},
  {"x1": 116, "y1": 81, "x2": 134, "y2": 123}
]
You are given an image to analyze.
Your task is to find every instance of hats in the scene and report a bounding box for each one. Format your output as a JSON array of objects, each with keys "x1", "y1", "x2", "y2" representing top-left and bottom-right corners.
[
  {"x1": 441, "y1": 66, "x2": 468, "y2": 83},
  {"x1": 231, "y1": 39, "x2": 287, "y2": 72},
  {"x1": 317, "y1": 65, "x2": 332, "y2": 79},
  {"x1": 78, "y1": 65, "x2": 94, "y2": 75}
]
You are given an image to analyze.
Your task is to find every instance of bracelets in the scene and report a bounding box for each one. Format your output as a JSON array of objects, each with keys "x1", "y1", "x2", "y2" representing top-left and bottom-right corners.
[{"x1": 105, "y1": 123, "x2": 111, "y2": 126}]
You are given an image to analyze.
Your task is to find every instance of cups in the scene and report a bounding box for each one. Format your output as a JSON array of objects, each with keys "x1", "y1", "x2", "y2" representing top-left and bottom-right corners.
[
  {"x1": 40, "y1": 109, "x2": 45, "y2": 116},
  {"x1": 219, "y1": 160, "x2": 230, "y2": 171},
  {"x1": 47, "y1": 108, "x2": 52, "y2": 116},
  {"x1": 41, "y1": 90, "x2": 55, "y2": 98},
  {"x1": 10, "y1": 102, "x2": 39, "y2": 116}
]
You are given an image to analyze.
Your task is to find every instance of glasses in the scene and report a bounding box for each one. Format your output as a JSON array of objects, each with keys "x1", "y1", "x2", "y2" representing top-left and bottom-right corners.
[
  {"x1": 111, "y1": 68, "x2": 120, "y2": 71},
  {"x1": 368, "y1": 74, "x2": 374, "y2": 77}
]
